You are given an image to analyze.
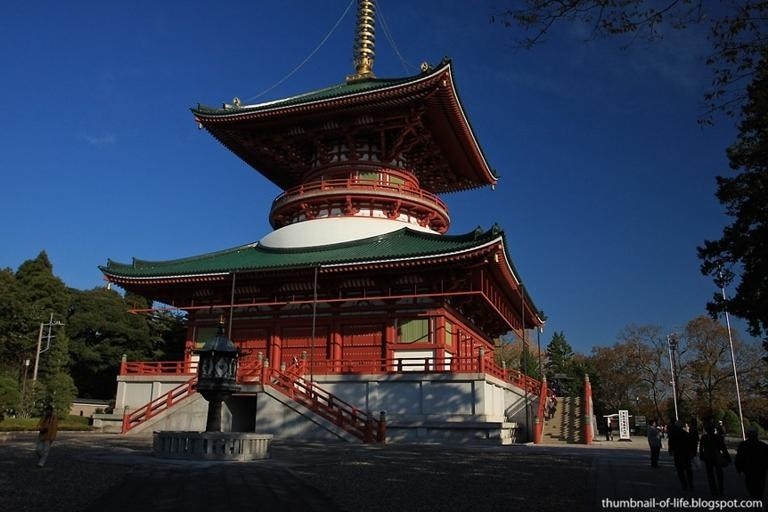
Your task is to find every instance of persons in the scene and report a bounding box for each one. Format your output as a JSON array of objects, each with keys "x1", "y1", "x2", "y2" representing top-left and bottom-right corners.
[
  {"x1": 36, "y1": 405, "x2": 58, "y2": 468},
  {"x1": 603, "y1": 423, "x2": 614, "y2": 441},
  {"x1": 544, "y1": 394, "x2": 558, "y2": 419},
  {"x1": 647, "y1": 418, "x2": 768, "y2": 501}
]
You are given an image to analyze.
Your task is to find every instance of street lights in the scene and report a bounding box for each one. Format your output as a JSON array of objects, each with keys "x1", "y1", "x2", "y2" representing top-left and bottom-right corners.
[{"x1": 20, "y1": 356, "x2": 33, "y2": 417}]
